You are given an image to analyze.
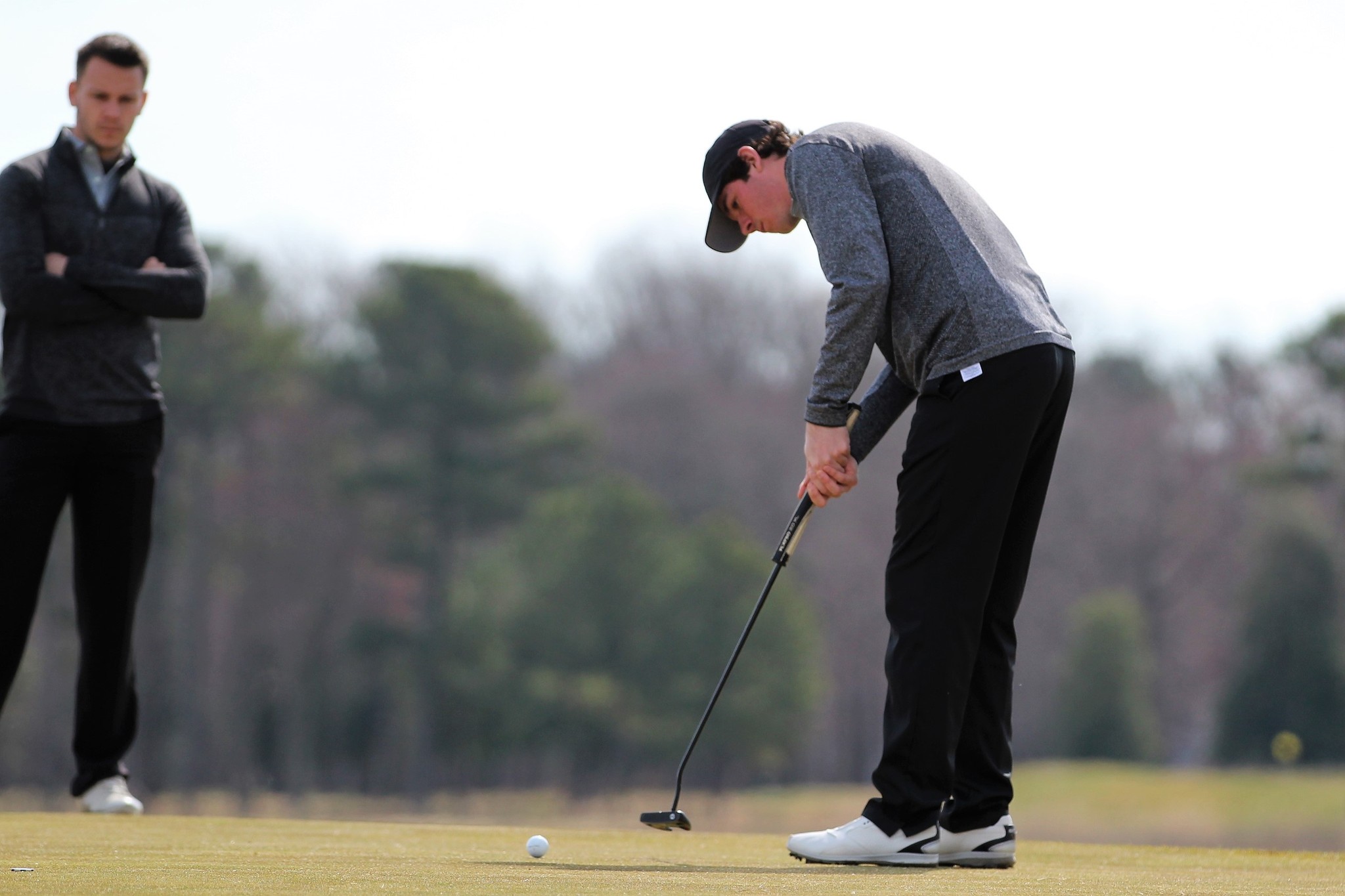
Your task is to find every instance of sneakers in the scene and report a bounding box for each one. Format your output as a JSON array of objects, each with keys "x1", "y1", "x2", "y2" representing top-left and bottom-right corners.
[
  {"x1": 788, "y1": 815, "x2": 938, "y2": 867},
  {"x1": 83, "y1": 777, "x2": 142, "y2": 815},
  {"x1": 938, "y1": 814, "x2": 1016, "y2": 870}
]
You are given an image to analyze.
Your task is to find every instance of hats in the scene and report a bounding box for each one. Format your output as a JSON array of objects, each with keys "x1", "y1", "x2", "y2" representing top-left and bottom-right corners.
[{"x1": 703, "y1": 120, "x2": 770, "y2": 253}]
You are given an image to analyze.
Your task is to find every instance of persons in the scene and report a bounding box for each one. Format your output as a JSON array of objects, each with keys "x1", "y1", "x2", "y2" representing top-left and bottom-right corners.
[
  {"x1": 703, "y1": 120, "x2": 1076, "y2": 870},
  {"x1": 0, "y1": 33, "x2": 208, "y2": 815}
]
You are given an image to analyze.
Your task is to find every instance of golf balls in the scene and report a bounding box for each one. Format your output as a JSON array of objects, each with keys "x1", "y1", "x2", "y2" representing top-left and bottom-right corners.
[{"x1": 526, "y1": 835, "x2": 549, "y2": 858}]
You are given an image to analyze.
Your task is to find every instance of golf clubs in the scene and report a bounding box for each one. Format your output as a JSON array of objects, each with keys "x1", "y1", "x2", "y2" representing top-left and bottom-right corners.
[{"x1": 635, "y1": 398, "x2": 864, "y2": 833}]
